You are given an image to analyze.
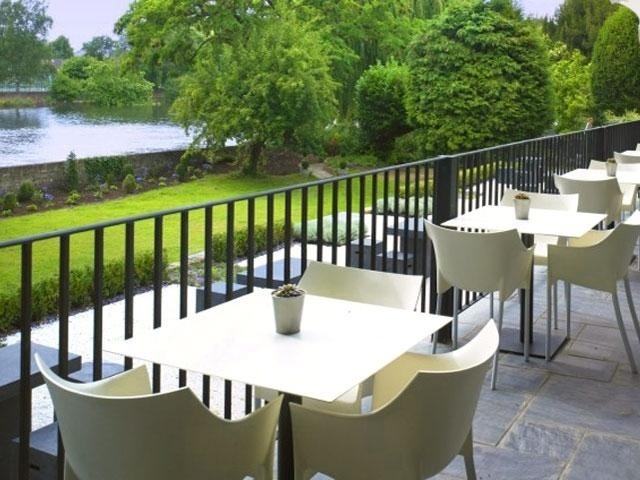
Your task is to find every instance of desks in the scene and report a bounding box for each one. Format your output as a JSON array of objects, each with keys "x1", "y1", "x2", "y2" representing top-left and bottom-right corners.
[
  {"x1": 0, "y1": 341, "x2": 82, "y2": 480},
  {"x1": 100, "y1": 286, "x2": 456, "y2": 480},
  {"x1": 438, "y1": 203, "x2": 611, "y2": 361}
]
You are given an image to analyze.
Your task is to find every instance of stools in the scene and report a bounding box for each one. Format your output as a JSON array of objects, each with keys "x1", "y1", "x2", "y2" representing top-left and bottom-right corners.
[{"x1": 10, "y1": 422, "x2": 57, "y2": 480}]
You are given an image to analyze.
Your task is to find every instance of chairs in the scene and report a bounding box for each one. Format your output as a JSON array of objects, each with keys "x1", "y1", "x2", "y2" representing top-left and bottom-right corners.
[
  {"x1": 34, "y1": 144, "x2": 640, "y2": 480},
  {"x1": 417, "y1": 218, "x2": 538, "y2": 390},
  {"x1": 488, "y1": 187, "x2": 570, "y2": 330},
  {"x1": 35, "y1": 351, "x2": 287, "y2": 480},
  {"x1": 541, "y1": 221, "x2": 638, "y2": 377},
  {"x1": 255, "y1": 259, "x2": 425, "y2": 427},
  {"x1": 287, "y1": 317, "x2": 503, "y2": 478}
]
